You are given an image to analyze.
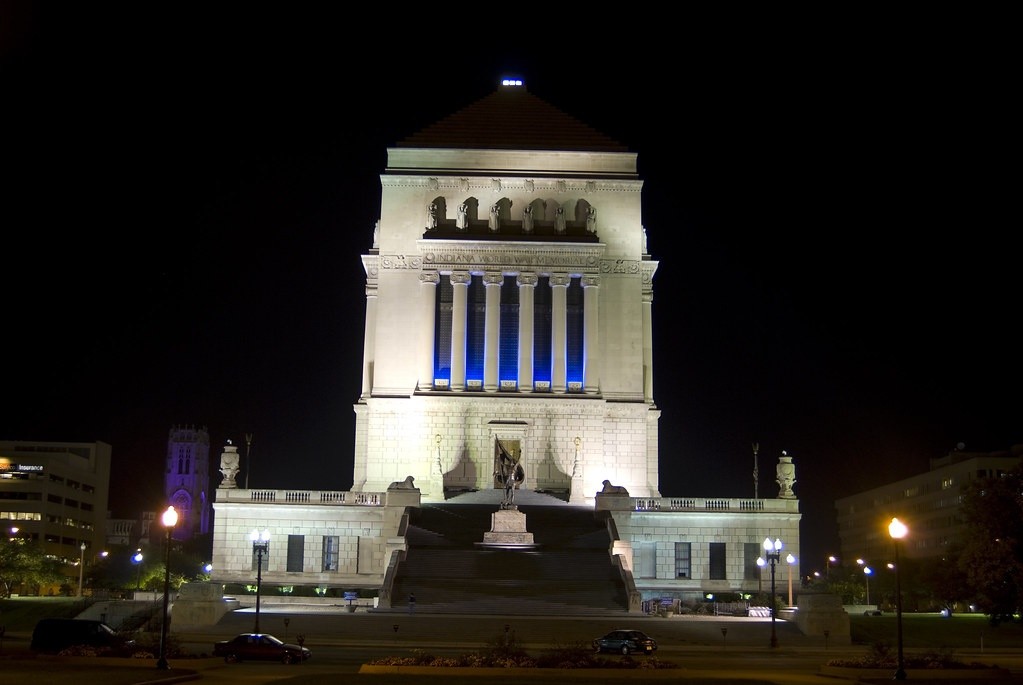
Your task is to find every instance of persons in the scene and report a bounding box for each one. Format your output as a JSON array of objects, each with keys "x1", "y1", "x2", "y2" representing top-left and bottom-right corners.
[
  {"x1": 408, "y1": 592, "x2": 417, "y2": 616},
  {"x1": 499, "y1": 449, "x2": 522, "y2": 505}
]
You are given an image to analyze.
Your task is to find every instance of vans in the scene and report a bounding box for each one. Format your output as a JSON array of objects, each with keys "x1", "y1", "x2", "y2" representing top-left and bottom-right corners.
[{"x1": 29, "y1": 617, "x2": 135, "y2": 659}]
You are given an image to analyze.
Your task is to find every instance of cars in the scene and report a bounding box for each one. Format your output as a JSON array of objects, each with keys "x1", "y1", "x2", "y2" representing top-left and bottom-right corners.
[
  {"x1": 213, "y1": 633, "x2": 313, "y2": 665},
  {"x1": 590, "y1": 629, "x2": 658, "y2": 657}
]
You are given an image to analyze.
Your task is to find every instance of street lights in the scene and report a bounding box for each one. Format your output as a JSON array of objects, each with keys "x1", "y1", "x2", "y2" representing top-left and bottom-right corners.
[
  {"x1": 889, "y1": 518, "x2": 908, "y2": 683},
  {"x1": 855, "y1": 558, "x2": 871, "y2": 605},
  {"x1": 205, "y1": 564, "x2": 212, "y2": 577},
  {"x1": 763, "y1": 537, "x2": 782, "y2": 648},
  {"x1": 76, "y1": 542, "x2": 86, "y2": 596},
  {"x1": 134, "y1": 553, "x2": 144, "y2": 591},
  {"x1": 249, "y1": 528, "x2": 270, "y2": 634},
  {"x1": 826, "y1": 556, "x2": 835, "y2": 577},
  {"x1": 786, "y1": 553, "x2": 795, "y2": 607},
  {"x1": 154, "y1": 506, "x2": 178, "y2": 668}
]
